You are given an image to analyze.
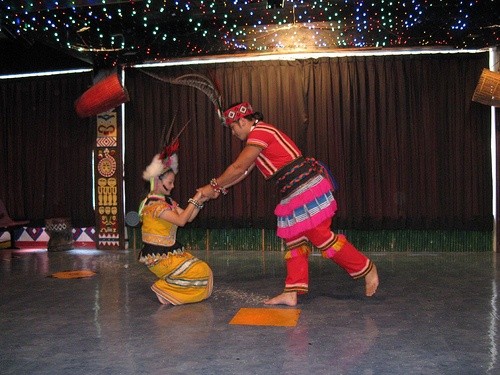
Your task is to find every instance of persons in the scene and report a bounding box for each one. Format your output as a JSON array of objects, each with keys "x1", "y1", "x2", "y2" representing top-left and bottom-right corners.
[
  {"x1": 196, "y1": 101, "x2": 379, "y2": 307},
  {"x1": 138, "y1": 138, "x2": 213, "y2": 305}
]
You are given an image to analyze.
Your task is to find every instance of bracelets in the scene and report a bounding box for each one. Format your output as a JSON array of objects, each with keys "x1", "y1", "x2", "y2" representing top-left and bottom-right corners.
[{"x1": 188, "y1": 178, "x2": 227, "y2": 209}]
